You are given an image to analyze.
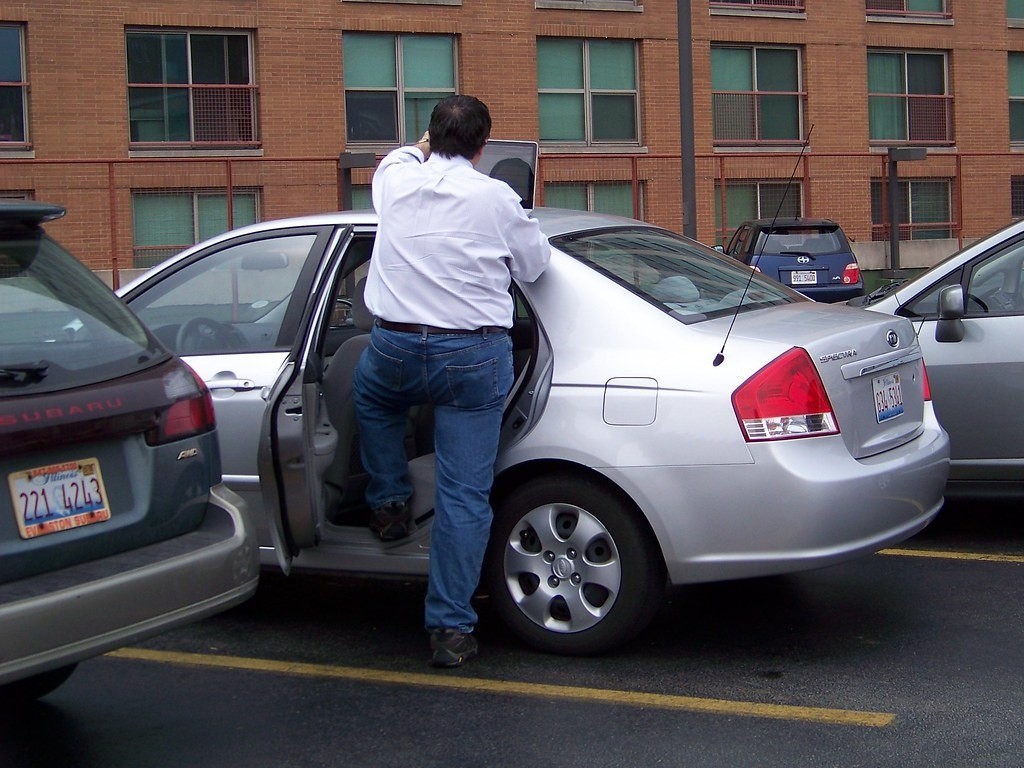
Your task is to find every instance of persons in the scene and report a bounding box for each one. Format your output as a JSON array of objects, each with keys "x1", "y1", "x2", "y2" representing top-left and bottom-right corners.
[
  {"x1": 351, "y1": 94, "x2": 551, "y2": 668},
  {"x1": 489, "y1": 157, "x2": 534, "y2": 209}
]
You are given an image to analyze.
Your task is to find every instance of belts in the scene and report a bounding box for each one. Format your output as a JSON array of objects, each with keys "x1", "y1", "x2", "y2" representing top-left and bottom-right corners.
[{"x1": 376, "y1": 318, "x2": 506, "y2": 335}]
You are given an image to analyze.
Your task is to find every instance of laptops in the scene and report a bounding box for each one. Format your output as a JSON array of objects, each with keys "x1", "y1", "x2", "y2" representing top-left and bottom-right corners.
[{"x1": 474, "y1": 139, "x2": 539, "y2": 216}]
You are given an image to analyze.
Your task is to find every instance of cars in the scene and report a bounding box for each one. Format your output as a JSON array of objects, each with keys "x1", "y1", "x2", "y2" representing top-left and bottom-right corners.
[
  {"x1": 709, "y1": 216, "x2": 865, "y2": 308},
  {"x1": 800, "y1": 216, "x2": 1023, "y2": 507},
  {"x1": 1, "y1": 198, "x2": 261, "y2": 703},
  {"x1": 45, "y1": 200, "x2": 949, "y2": 658}
]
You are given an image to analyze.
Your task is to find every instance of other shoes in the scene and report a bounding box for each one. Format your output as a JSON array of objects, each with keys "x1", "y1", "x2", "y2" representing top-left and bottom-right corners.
[
  {"x1": 367, "y1": 498, "x2": 411, "y2": 544},
  {"x1": 431, "y1": 628, "x2": 477, "y2": 667}
]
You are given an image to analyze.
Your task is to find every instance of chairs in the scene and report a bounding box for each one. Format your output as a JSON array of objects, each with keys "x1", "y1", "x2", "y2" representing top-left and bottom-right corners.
[
  {"x1": 763, "y1": 241, "x2": 787, "y2": 252},
  {"x1": 803, "y1": 239, "x2": 834, "y2": 250},
  {"x1": 312, "y1": 275, "x2": 372, "y2": 482}
]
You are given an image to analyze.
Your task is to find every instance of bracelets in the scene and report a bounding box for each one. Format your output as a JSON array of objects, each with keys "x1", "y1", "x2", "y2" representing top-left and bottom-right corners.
[{"x1": 416, "y1": 136, "x2": 430, "y2": 144}]
